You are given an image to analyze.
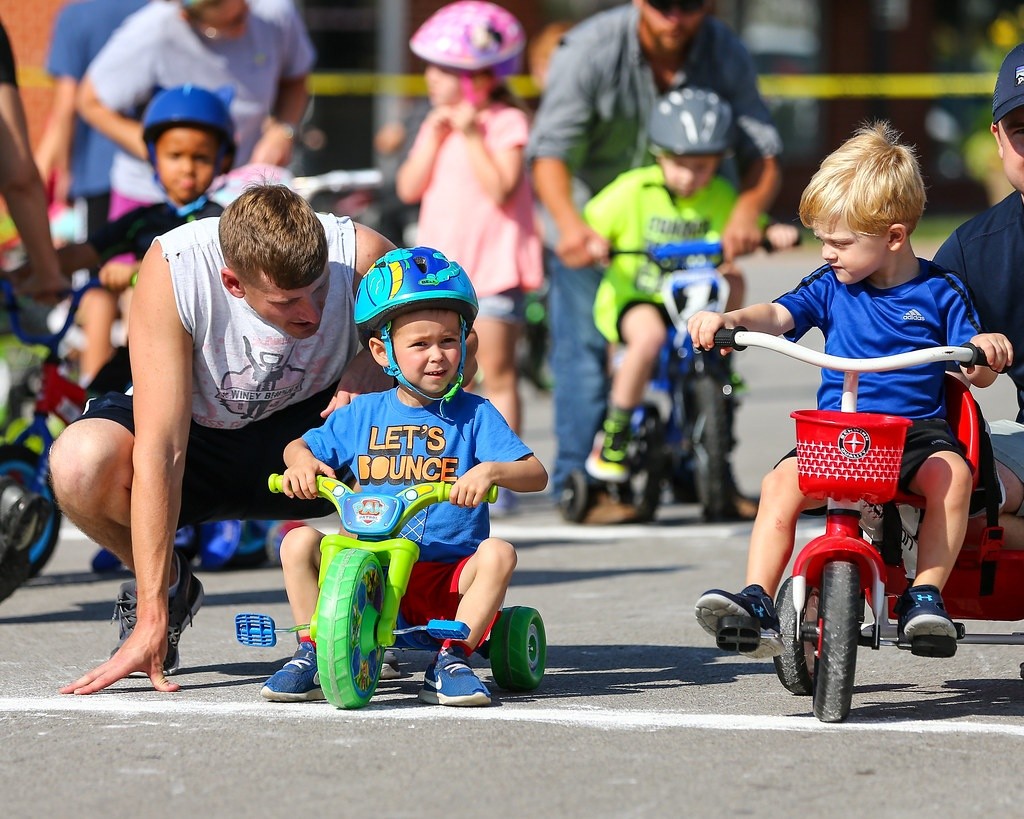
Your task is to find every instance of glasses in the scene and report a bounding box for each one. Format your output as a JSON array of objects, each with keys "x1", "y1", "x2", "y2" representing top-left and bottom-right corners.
[{"x1": 647, "y1": 0, "x2": 703, "y2": 13}]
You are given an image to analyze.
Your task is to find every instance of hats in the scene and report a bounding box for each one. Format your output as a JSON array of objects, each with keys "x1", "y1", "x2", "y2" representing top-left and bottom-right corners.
[{"x1": 993, "y1": 44, "x2": 1024, "y2": 125}]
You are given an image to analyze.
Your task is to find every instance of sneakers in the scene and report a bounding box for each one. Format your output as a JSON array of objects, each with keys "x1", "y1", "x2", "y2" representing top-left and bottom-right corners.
[
  {"x1": 379, "y1": 652, "x2": 401, "y2": 679},
  {"x1": 894, "y1": 585, "x2": 957, "y2": 643},
  {"x1": 261, "y1": 642, "x2": 325, "y2": 701},
  {"x1": 112, "y1": 548, "x2": 204, "y2": 677},
  {"x1": 418, "y1": 651, "x2": 491, "y2": 706},
  {"x1": 695, "y1": 585, "x2": 784, "y2": 659},
  {"x1": 584, "y1": 410, "x2": 632, "y2": 483}
]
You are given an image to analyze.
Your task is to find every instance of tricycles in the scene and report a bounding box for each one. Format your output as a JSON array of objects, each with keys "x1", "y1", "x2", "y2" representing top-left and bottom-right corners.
[
  {"x1": 696, "y1": 328, "x2": 1024, "y2": 724},
  {"x1": 235, "y1": 472, "x2": 548, "y2": 710}
]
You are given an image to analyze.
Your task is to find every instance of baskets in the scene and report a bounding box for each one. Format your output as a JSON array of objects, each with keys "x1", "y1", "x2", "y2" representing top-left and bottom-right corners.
[{"x1": 791, "y1": 410, "x2": 914, "y2": 505}]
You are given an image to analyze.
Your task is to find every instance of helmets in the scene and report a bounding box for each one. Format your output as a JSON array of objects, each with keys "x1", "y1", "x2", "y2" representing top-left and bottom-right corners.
[
  {"x1": 411, "y1": 0, "x2": 527, "y2": 69},
  {"x1": 142, "y1": 82, "x2": 234, "y2": 142},
  {"x1": 354, "y1": 246, "x2": 479, "y2": 353},
  {"x1": 651, "y1": 89, "x2": 733, "y2": 156}
]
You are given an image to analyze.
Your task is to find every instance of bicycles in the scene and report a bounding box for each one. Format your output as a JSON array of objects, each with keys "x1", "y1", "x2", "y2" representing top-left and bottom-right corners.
[
  {"x1": 553, "y1": 230, "x2": 805, "y2": 526},
  {"x1": 0, "y1": 264, "x2": 140, "y2": 606}
]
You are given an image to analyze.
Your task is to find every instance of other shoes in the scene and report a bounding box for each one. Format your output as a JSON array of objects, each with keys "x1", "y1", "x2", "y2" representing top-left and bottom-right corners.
[
  {"x1": 733, "y1": 491, "x2": 757, "y2": 517},
  {"x1": 557, "y1": 487, "x2": 640, "y2": 524},
  {"x1": 487, "y1": 488, "x2": 518, "y2": 516},
  {"x1": 91, "y1": 528, "x2": 195, "y2": 571},
  {"x1": 191, "y1": 521, "x2": 272, "y2": 570}
]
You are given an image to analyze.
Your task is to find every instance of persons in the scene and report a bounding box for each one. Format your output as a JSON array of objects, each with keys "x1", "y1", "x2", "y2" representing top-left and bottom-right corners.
[
  {"x1": 933, "y1": 44, "x2": 1024, "y2": 552},
  {"x1": 0, "y1": 0, "x2": 784, "y2": 574},
  {"x1": 260, "y1": 246, "x2": 551, "y2": 706},
  {"x1": 686, "y1": 121, "x2": 1014, "y2": 638},
  {"x1": 45, "y1": 171, "x2": 401, "y2": 696}
]
